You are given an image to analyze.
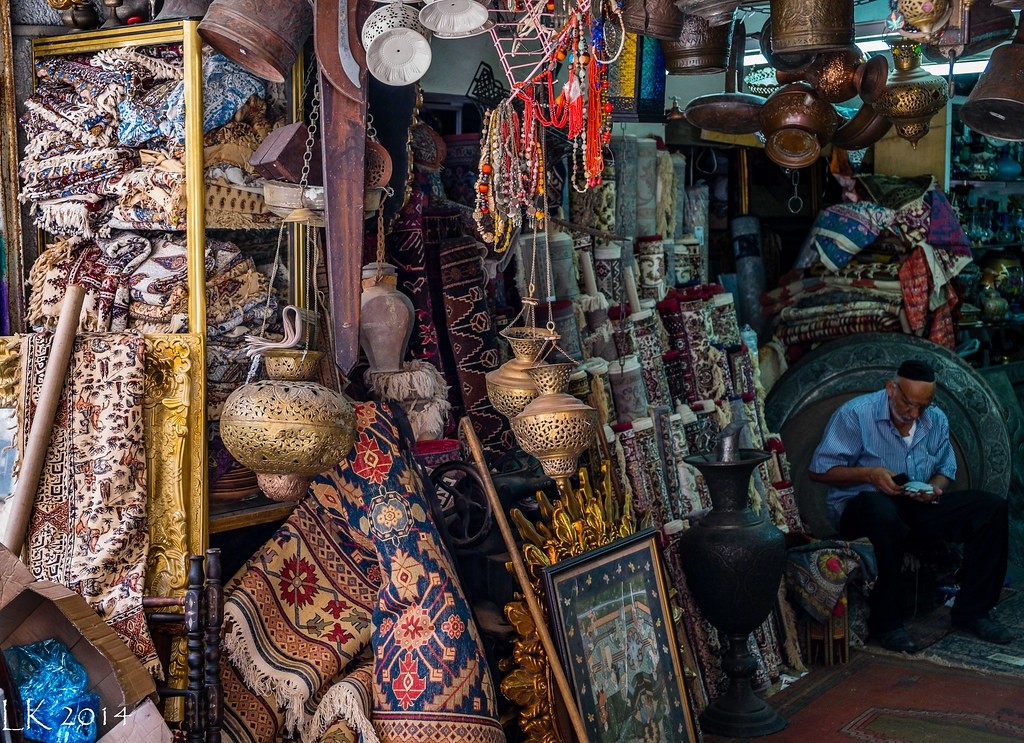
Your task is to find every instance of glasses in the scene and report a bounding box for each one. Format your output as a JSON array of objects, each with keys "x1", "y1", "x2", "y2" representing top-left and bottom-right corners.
[{"x1": 894, "y1": 383, "x2": 929, "y2": 411}]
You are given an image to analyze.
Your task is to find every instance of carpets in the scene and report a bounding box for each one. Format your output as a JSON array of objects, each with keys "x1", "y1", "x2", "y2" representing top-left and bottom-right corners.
[{"x1": 701, "y1": 645, "x2": 1024, "y2": 743}]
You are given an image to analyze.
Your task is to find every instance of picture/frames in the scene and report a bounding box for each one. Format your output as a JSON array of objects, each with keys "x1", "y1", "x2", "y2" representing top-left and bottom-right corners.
[{"x1": 539, "y1": 526, "x2": 699, "y2": 743}]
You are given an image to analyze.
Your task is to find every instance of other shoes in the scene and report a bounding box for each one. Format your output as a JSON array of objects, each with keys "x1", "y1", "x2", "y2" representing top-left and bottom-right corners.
[
  {"x1": 867, "y1": 624, "x2": 918, "y2": 652},
  {"x1": 951, "y1": 611, "x2": 1012, "y2": 644}
]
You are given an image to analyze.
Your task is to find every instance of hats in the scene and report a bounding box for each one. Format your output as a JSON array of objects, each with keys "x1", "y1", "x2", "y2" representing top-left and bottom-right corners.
[{"x1": 897, "y1": 360, "x2": 935, "y2": 381}]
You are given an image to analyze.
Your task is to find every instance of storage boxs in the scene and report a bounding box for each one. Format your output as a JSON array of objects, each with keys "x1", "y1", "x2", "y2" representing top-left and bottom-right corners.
[{"x1": 0, "y1": 542, "x2": 174, "y2": 743}]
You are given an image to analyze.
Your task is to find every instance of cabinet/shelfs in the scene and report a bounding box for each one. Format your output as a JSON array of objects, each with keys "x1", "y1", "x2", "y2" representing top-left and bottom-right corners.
[
  {"x1": 874, "y1": 95, "x2": 1024, "y2": 369},
  {"x1": 32, "y1": 20, "x2": 305, "y2": 721}
]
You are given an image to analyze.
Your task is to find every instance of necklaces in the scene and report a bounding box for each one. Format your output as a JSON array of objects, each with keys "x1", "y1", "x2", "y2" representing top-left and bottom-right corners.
[{"x1": 472, "y1": 0, "x2": 625, "y2": 253}]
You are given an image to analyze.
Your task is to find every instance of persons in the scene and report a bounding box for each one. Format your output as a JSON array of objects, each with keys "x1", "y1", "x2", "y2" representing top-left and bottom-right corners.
[{"x1": 807, "y1": 360, "x2": 1014, "y2": 651}]
